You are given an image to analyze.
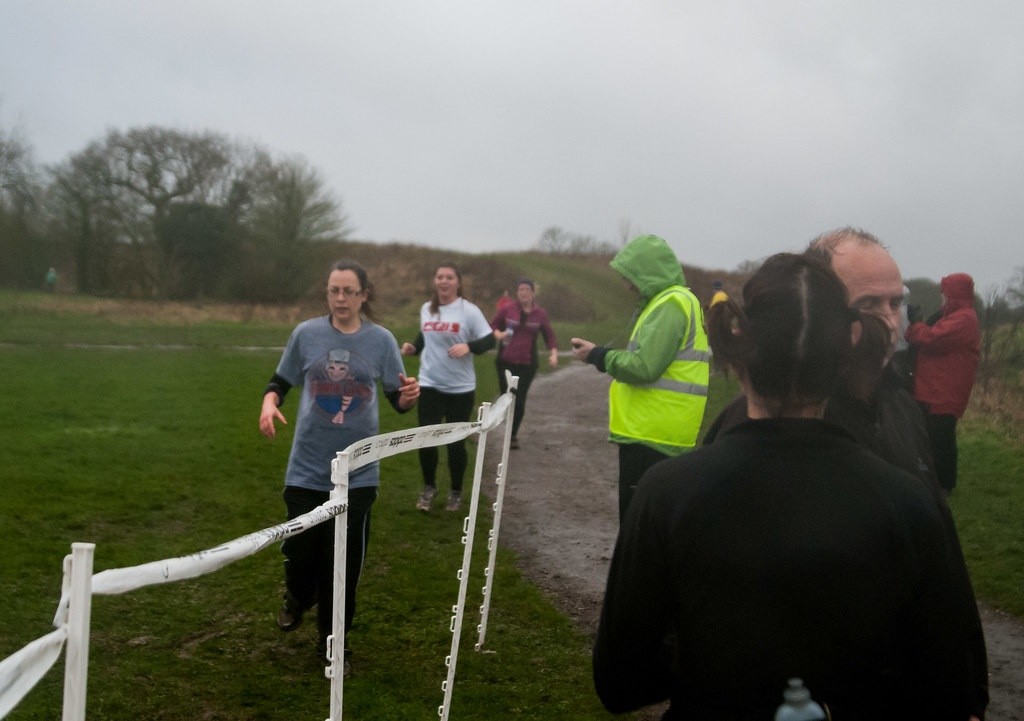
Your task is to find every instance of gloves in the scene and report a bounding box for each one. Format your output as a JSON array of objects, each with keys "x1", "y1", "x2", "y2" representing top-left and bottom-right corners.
[{"x1": 906, "y1": 304, "x2": 922, "y2": 324}]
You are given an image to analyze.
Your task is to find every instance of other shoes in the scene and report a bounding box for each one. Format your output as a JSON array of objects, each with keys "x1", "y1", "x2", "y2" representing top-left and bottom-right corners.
[
  {"x1": 277, "y1": 606, "x2": 303, "y2": 631},
  {"x1": 510, "y1": 435, "x2": 518, "y2": 448},
  {"x1": 444, "y1": 487, "x2": 463, "y2": 512},
  {"x1": 415, "y1": 486, "x2": 439, "y2": 513},
  {"x1": 320, "y1": 642, "x2": 351, "y2": 656}
]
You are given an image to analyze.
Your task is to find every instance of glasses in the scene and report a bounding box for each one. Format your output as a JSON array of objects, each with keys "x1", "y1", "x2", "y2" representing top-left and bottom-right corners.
[{"x1": 326, "y1": 282, "x2": 366, "y2": 297}]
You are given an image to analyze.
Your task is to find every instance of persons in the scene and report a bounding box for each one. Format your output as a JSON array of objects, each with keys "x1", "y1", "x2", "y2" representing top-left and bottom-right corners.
[
  {"x1": 588, "y1": 248, "x2": 991, "y2": 721},
  {"x1": 399, "y1": 261, "x2": 496, "y2": 514},
  {"x1": 259, "y1": 262, "x2": 423, "y2": 682},
  {"x1": 904, "y1": 272, "x2": 983, "y2": 493},
  {"x1": 704, "y1": 226, "x2": 935, "y2": 483},
  {"x1": 490, "y1": 280, "x2": 559, "y2": 451},
  {"x1": 495, "y1": 288, "x2": 513, "y2": 312},
  {"x1": 570, "y1": 233, "x2": 709, "y2": 528}
]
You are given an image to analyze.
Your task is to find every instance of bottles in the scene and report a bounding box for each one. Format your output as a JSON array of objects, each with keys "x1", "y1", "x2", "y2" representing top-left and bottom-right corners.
[
  {"x1": 775, "y1": 678, "x2": 825, "y2": 721},
  {"x1": 502, "y1": 326, "x2": 514, "y2": 344}
]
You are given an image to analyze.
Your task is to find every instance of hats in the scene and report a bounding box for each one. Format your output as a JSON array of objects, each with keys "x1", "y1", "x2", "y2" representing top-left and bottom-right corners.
[{"x1": 518, "y1": 278, "x2": 534, "y2": 292}]
